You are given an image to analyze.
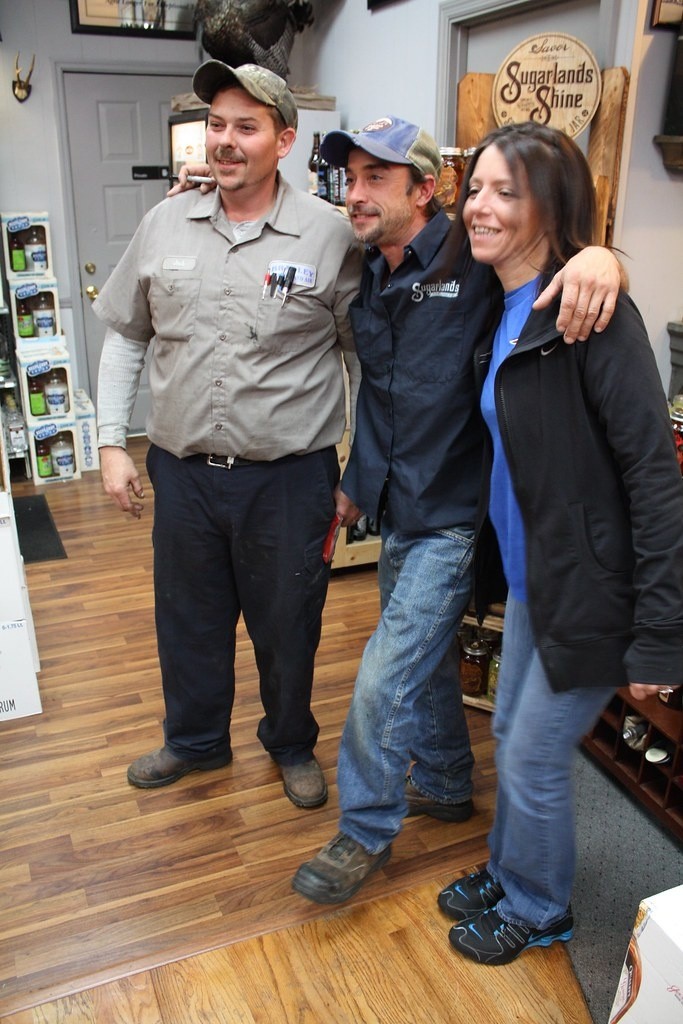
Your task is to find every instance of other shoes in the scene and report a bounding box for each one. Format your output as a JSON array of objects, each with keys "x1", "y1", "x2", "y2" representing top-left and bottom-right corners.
[
  {"x1": 289, "y1": 829, "x2": 392, "y2": 906},
  {"x1": 399, "y1": 779, "x2": 472, "y2": 825},
  {"x1": 124, "y1": 740, "x2": 234, "y2": 789},
  {"x1": 275, "y1": 752, "x2": 329, "y2": 808}
]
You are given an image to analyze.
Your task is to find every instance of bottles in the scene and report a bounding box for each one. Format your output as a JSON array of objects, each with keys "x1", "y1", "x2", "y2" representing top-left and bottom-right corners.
[
  {"x1": 306, "y1": 130, "x2": 349, "y2": 207},
  {"x1": 0, "y1": 228, "x2": 75, "y2": 477},
  {"x1": 432, "y1": 146, "x2": 474, "y2": 213},
  {"x1": 454, "y1": 623, "x2": 502, "y2": 703}
]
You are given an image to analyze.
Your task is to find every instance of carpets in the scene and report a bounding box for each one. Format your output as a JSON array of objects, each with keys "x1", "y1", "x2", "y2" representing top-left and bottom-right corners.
[{"x1": 12, "y1": 495, "x2": 68, "y2": 564}]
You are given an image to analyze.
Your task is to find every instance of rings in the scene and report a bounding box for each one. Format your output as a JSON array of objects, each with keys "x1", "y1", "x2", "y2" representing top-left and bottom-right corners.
[{"x1": 658, "y1": 684, "x2": 674, "y2": 694}]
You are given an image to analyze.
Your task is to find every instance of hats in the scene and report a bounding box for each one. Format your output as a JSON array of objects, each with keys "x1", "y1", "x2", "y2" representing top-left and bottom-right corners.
[
  {"x1": 193, "y1": 58, "x2": 300, "y2": 133},
  {"x1": 320, "y1": 116, "x2": 444, "y2": 189}
]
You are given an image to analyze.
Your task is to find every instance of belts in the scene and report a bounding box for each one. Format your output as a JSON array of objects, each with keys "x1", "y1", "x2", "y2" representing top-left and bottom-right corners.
[{"x1": 193, "y1": 453, "x2": 256, "y2": 469}]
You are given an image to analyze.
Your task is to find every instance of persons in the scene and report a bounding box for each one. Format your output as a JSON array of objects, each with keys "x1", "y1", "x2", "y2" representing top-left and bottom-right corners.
[
  {"x1": 167, "y1": 115, "x2": 628, "y2": 906},
  {"x1": 92, "y1": 58, "x2": 368, "y2": 808},
  {"x1": 435, "y1": 125, "x2": 683, "y2": 965}
]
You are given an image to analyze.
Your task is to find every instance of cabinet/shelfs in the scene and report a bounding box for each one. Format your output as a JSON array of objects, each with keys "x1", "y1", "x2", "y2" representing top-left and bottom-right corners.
[
  {"x1": 0, "y1": 212, "x2": 80, "y2": 485},
  {"x1": 584, "y1": 684, "x2": 683, "y2": 843}
]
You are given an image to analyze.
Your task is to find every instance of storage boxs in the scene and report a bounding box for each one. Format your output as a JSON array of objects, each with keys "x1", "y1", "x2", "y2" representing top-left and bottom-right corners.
[{"x1": 72, "y1": 389, "x2": 100, "y2": 472}]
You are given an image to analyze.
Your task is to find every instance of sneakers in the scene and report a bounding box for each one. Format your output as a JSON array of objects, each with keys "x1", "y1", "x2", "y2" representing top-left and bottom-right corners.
[
  {"x1": 436, "y1": 869, "x2": 506, "y2": 921},
  {"x1": 447, "y1": 903, "x2": 576, "y2": 968}
]
